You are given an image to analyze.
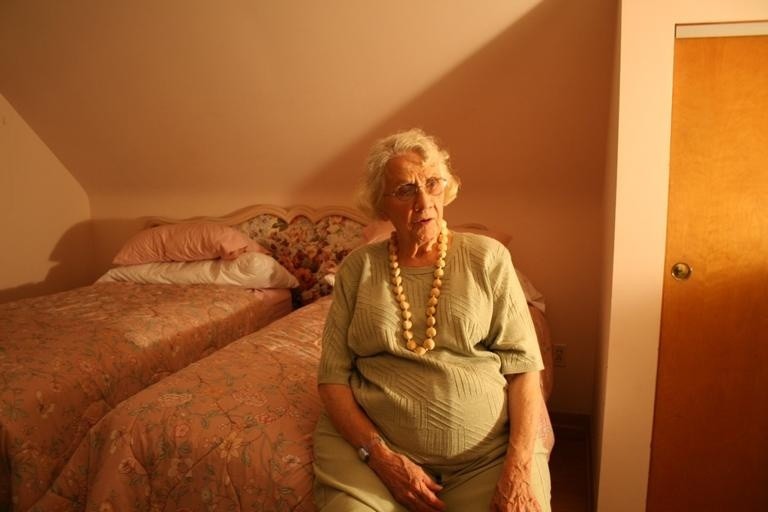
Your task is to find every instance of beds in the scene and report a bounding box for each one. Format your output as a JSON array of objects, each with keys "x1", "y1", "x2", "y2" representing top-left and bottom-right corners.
[
  {"x1": 67, "y1": 285, "x2": 548, "y2": 512},
  {"x1": 3, "y1": 282, "x2": 291, "y2": 512}
]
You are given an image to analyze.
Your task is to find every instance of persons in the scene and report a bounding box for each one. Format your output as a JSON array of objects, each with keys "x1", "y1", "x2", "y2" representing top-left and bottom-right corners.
[{"x1": 310, "y1": 126, "x2": 555, "y2": 512}]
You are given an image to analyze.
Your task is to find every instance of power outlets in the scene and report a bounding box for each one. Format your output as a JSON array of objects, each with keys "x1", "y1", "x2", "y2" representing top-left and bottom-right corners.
[{"x1": 551, "y1": 341, "x2": 571, "y2": 369}]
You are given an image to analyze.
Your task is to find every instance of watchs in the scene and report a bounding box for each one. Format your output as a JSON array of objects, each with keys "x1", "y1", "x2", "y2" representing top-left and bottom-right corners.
[{"x1": 358, "y1": 437, "x2": 384, "y2": 464}]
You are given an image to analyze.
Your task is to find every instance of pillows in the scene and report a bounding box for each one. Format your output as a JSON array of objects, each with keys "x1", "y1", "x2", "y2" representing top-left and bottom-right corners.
[
  {"x1": 89, "y1": 252, "x2": 300, "y2": 289},
  {"x1": 111, "y1": 221, "x2": 267, "y2": 264}
]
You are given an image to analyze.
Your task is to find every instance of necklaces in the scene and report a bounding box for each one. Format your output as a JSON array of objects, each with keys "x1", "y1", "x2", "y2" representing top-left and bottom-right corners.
[{"x1": 388, "y1": 219, "x2": 449, "y2": 355}]
